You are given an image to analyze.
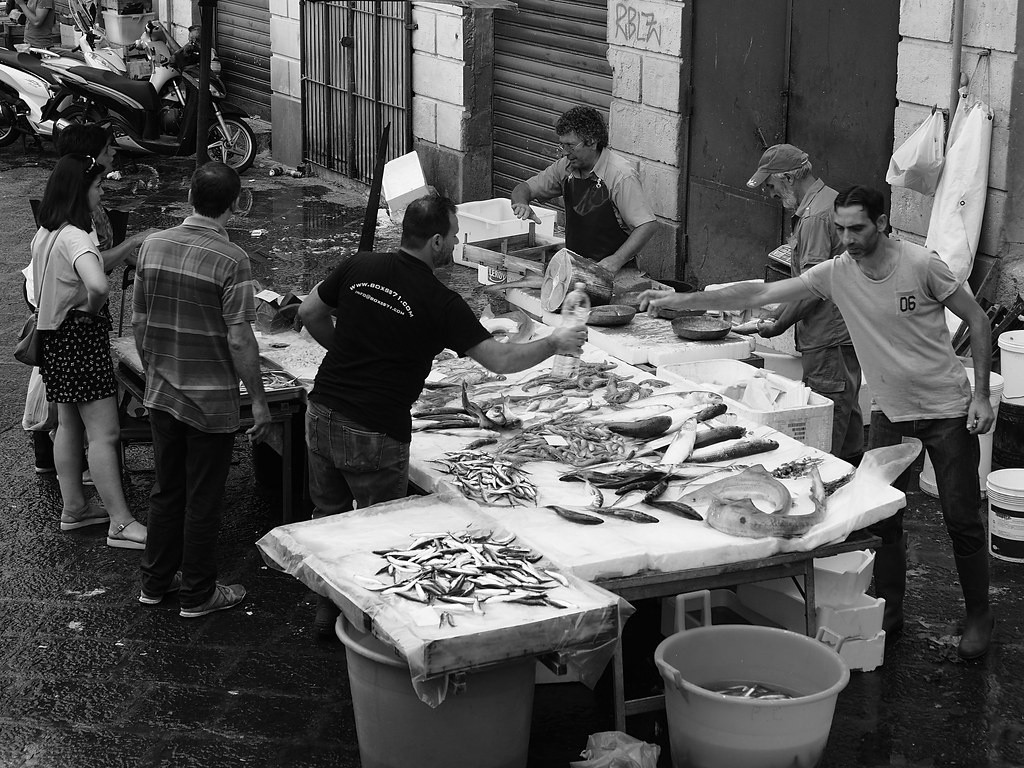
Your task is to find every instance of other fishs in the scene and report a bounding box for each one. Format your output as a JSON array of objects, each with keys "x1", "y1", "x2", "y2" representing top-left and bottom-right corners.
[
  {"x1": 475, "y1": 248, "x2": 647, "y2": 313},
  {"x1": 412, "y1": 352, "x2": 827, "y2": 536},
  {"x1": 479, "y1": 304, "x2": 539, "y2": 344},
  {"x1": 366, "y1": 522, "x2": 568, "y2": 628}
]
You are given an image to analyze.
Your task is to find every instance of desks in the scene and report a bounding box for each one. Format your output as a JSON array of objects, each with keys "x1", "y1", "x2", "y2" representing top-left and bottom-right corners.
[{"x1": 111, "y1": 333, "x2": 304, "y2": 524}]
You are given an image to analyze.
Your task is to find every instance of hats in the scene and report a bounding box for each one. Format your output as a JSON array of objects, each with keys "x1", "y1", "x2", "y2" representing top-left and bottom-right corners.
[{"x1": 747, "y1": 144, "x2": 809, "y2": 189}]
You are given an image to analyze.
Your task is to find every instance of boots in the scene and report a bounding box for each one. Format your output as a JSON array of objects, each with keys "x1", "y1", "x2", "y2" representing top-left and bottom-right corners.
[
  {"x1": 875, "y1": 536, "x2": 908, "y2": 637},
  {"x1": 955, "y1": 544, "x2": 997, "y2": 658}
]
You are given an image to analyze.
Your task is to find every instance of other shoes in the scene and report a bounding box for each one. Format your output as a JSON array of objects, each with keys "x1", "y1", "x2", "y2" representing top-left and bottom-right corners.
[
  {"x1": 314, "y1": 608, "x2": 340, "y2": 636},
  {"x1": 34, "y1": 466, "x2": 53, "y2": 473},
  {"x1": 82, "y1": 469, "x2": 95, "y2": 484}
]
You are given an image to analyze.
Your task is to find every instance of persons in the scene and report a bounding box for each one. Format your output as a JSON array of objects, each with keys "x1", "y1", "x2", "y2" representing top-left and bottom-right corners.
[
  {"x1": 511, "y1": 107, "x2": 659, "y2": 278},
  {"x1": 130, "y1": 162, "x2": 272, "y2": 618},
  {"x1": 637, "y1": 185, "x2": 996, "y2": 660},
  {"x1": 20, "y1": 124, "x2": 163, "y2": 549},
  {"x1": 14, "y1": 0, "x2": 55, "y2": 59},
  {"x1": 747, "y1": 144, "x2": 865, "y2": 467},
  {"x1": 298, "y1": 195, "x2": 589, "y2": 644}
]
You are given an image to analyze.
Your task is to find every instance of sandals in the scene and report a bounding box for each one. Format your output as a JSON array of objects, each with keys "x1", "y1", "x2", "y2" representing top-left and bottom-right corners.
[
  {"x1": 107, "y1": 519, "x2": 148, "y2": 550},
  {"x1": 61, "y1": 505, "x2": 109, "y2": 532}
]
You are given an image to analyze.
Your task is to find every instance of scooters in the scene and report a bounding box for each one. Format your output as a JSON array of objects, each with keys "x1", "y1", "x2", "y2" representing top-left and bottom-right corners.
[
  {"x1": 38, "y1": 36, "x2": 259, "y2": 176},
  {"x1": 0, "y1": 0, "x2": 195, "y2": 157}
]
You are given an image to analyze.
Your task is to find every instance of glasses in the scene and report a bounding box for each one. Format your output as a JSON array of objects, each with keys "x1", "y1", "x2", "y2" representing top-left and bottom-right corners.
[{"x1": 559, "y1": 141, "x2": 583, "y2": 154}]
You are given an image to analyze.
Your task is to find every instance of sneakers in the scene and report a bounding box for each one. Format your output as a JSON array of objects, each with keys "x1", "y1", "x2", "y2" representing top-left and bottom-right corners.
[
  {"x1": 179, "y1": 581, "x2": 246, "y2": 619},
  {"x1": 140, "y1": 571, "x2": 182, "y2": 605}
]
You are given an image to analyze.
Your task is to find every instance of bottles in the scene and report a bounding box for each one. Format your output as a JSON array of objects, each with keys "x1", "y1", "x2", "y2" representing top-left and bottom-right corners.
[
  {"x1": 299, "y1": 161, "x2": 305, "y2": 178},
  {"x1": 306, "y1": 161, "x2": 311, "y2": 178},
  {"x1": 551, "y1": 282, "x2": 591, "y2": 378}
]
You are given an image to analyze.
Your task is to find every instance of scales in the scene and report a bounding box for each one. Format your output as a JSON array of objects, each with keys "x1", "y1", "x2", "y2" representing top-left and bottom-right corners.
[{"x1": 767, "y1": 241, "x2": 791, "y2": 275}]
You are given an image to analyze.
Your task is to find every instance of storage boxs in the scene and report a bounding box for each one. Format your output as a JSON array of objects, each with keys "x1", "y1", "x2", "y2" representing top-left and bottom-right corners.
[
  {"x1": 107, "y1": 0, "x2": 153, "y2": 16},
  {"x1": 828, "y1": 629, "x2": 887, "y2": 673},
  {"x1": 109, "y1": 43, "x2": 146, "y2": 57},
  {"x1": 658, "y1": 586, "x2": 790, "y2": 637},
  {"x1": 100, "y1": 11, "x2": 156, "y2": 46},
  {"x1": 478, "y1": 262, "x2": 507, "y2": 293},
  {"x1": 655, "y1": 357, "x2": 835, "y2": 453},
  {"x1": 751, "y1": 548, "x2": 876, "y2": 606},
  {"x1": 125, "y1": 56, "x2": 153, "y2": 79},
  {"x1": 454, "y1": 197, "x2": 559, "y2": 269},
  {"x1": 59, "y1": 22, "x2": 82, "y2": 48},
  {"x1": 461, "y1": 223, "x2": 566, "y2": 276},
  {"x1": 735, "y1": 583, "x2": 887, "y2": 641},
  {"x1": 3, "y1": 22, "x2": 25, "y2": 36}
]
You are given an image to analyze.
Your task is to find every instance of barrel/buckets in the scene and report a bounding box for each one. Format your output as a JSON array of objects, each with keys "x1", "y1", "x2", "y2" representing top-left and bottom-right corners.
[
  {"x1": 335, "y1": 612, "x2": 536, "y2": 768},
  {"x1": 997, "y1": 330, "x2": 1024, "y2": 406},
  {"x1": 654, "y1": 591, "x2": 850, "y2": 768},
  {"x1": 985, "y1": 468, "x2": 1024, "y2": 563},
  {"x1": 920, "y1": 367, "x2": 1006, "y2": 498},
  {"x1": 653, "y1": 278, "x2": 697, "y2": 294}
]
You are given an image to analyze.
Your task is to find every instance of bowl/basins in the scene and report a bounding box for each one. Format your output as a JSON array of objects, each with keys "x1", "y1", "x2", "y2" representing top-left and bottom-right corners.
[
  {"x1": 659, "y1": 309, "x2": 708, "y2": 321},
  {"x1": 671, "y1": 315, "x2": 731, "y2": 340},
  {"x1": 590, "y1": 305, "x2": 637, "y2": 325}
]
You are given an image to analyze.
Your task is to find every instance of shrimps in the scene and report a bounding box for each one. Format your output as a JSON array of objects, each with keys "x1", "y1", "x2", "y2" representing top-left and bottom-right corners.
[{"x1": 521, "y1": 358, "x2": 673, "y2": 413}]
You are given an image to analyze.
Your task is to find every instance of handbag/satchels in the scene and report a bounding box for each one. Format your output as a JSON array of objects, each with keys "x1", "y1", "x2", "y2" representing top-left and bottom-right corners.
[
  {"x1": 23, "y1": 366, "x2": 58, "y2": 431},
  {"x1": 29, "y1": 199, "x2": 129, "y2": 248},
  {"x1": 13, "y1": 314, "x2": 38, "y2": 367}
]
